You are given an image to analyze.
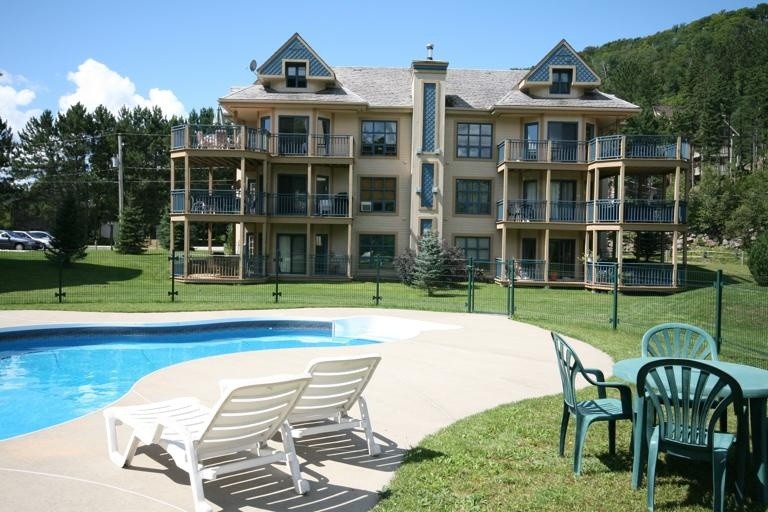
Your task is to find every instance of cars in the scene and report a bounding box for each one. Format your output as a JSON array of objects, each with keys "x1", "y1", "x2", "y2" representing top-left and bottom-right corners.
[{"x1": 0, "y1": 229, "x2": 55, "y2": 251}]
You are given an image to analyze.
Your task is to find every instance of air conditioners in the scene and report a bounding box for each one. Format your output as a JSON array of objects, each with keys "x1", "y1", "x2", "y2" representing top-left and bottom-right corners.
[{"x1": 360, "y1": 201, "x2": 372, "y2": 212}]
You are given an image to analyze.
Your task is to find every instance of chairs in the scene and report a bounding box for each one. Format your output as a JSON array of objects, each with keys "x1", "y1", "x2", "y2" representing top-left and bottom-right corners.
[
  {"x1": 639, "y1": 324, "x2": 730, "y2": 435},
  {"x1": 552, "y1": 328, "x2": 639, "y2": 476},
  {"x1": 102, "y1": 376, "x2": 313, "y2": 512},
  {"x1": 219, "y1": 354, "x2": 382, "y2": 466},
  {"x1": 295, "y1": 199, "x2": 333, "y2": 217},
  {"x1": 194, "y1": 129, "x2": 250, "y2": 151},
  {"x1": 190, "y1": 195, "x2": 206, "y2": 213}
]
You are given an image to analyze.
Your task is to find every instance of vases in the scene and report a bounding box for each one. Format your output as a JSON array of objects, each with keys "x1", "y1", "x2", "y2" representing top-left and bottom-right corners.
[{"x1": 549, "y1": 271, "x2": 557, "y2": 280}]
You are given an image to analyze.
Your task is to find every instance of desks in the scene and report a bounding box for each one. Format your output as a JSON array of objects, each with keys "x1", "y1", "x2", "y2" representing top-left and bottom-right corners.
[{"x1": 608, "y1": 354, "x2": 768, "y2": 507}]
